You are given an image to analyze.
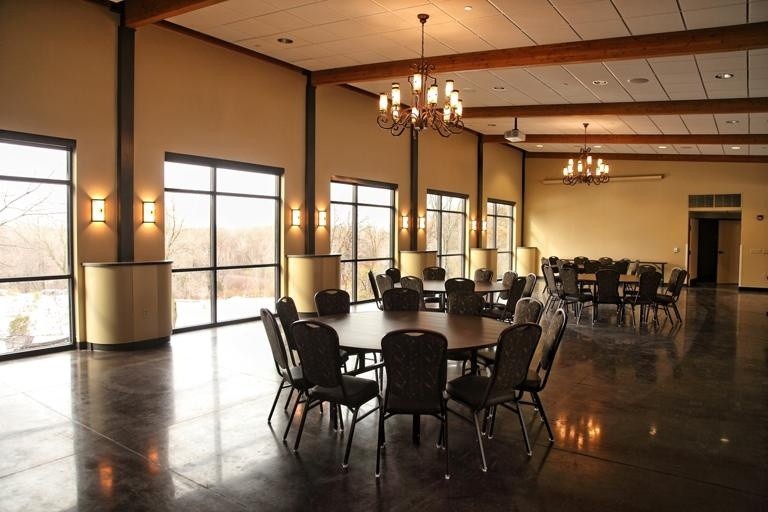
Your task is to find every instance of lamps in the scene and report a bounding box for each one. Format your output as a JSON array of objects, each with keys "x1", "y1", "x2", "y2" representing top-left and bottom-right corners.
[
  {"x1": 92, "y1": 199, "x2": 106, "y2": 220},
  {"x1": 376, "y1": 14, "x2": 464, "y2": 140},
  {"x1": 562, "y1": 124, "x2": 609, "y2": 187},
  {"x1": 418, "y1": 217, "x2": 426, "y2": 229},
  {"x1": 145, "y1": 201, "x2": 156, "y2": 222},
  {"x1": 292, "y1": 209, "x2": 301, "y2": 225},
  {"x1": 470, "y1": 219, "x2": 477, "y2": 230},
  {"x1": 484, "y1": 220, "x2": 488, "y2": 229},
  {"x1": 401, "y1": 216, "x2": 409, "y2": 229},
  {"x1": 318, "y1": 209, "x2": 327, "y2": 226}
]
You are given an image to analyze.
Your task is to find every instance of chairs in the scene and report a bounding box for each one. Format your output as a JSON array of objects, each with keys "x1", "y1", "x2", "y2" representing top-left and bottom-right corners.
[{"x1": 538, "y1": 258, "x2": 692, "y2": 331}]
